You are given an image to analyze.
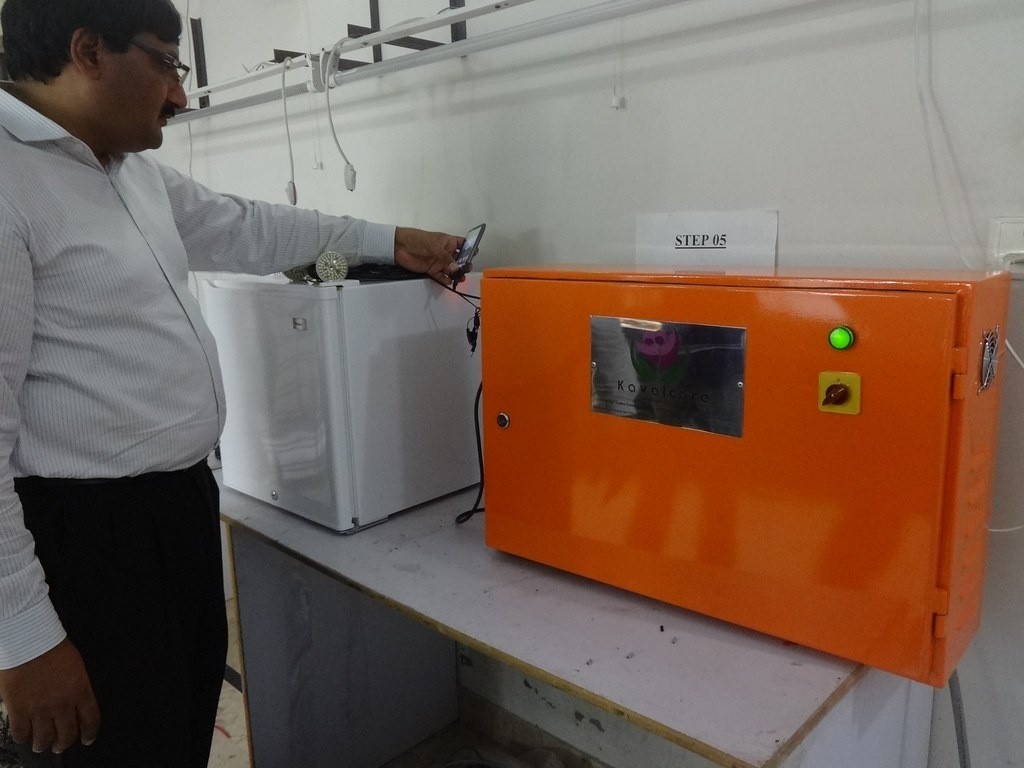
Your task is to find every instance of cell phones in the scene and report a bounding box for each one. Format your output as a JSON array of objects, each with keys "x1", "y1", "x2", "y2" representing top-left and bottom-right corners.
[{"x1": 450, "y1": 223, "x2": 487, "y2": 280}]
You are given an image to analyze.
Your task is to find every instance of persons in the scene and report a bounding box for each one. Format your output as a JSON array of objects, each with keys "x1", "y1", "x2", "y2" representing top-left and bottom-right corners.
[{"x1": 1, "y1": 1, "x2": 480, "y2": 768}]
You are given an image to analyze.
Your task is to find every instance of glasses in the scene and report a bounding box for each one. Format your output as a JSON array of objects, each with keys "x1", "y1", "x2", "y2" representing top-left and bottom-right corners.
[{"x1": 129, "y1": 38, "x2": 190, "y2": 89}]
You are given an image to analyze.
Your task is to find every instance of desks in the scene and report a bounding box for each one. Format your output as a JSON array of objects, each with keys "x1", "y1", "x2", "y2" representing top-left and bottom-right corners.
[{"x1": 211, "y1": 467, "x2": 933, "y2": 768}]
